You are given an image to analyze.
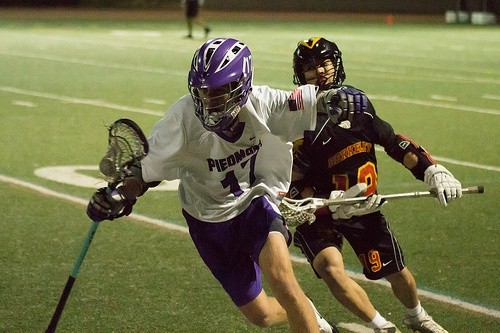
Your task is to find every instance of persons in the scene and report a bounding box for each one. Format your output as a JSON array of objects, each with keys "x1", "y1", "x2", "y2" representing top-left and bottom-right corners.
[
  {"x1": 180, "y1": 0, "x2": 212, "y2": 38},
  {"x1": 87, "y1": 38, "x2": 368, "y2": 333},
  {"x1": 284, "y1": 38, "x2": 463, "y2": 333}
]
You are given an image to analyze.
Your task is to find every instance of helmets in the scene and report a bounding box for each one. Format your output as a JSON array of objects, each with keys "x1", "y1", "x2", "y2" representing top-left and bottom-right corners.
[
  {"x1": 188, "y1": 37, "x2": 255, "y2": 131},
  {"x1": 292, "y1": 36, "x2": 346, "y2": 91}
]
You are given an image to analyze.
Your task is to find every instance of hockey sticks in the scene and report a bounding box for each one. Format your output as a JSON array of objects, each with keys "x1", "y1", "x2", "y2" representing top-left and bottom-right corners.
[
  {"x1": 279, "y1": 185, "x2": 484, "y2": 227},
  {"x1": 43, "y1": 118, "x2": 149, "y2": 333}
]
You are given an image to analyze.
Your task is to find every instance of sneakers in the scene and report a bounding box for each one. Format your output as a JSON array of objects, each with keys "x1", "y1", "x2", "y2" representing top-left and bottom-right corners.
[
  {"x1": 304, "y1": 292, "x2": 340, "y2": 333},
  {"x1": 374, "y1": 321, "x2": 402, "y2": 333},
  {"x1": 402, "y1": 306, "x2": 449, "y2": 333}
]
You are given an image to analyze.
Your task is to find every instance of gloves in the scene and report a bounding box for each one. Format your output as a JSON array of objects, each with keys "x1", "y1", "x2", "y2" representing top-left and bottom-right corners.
[
  {"x1": 86, "y1": 187, "x2": 129, "y2": 222},
  {"x1": 328, "y1": 183, "x2": 388, "y2": 219},
  {"x1": 326, "y1": 86, "x2": 367, "y2": 125},
  {"x1": 424, "y1": 164, "x2": 463, "y2": 207}
]
